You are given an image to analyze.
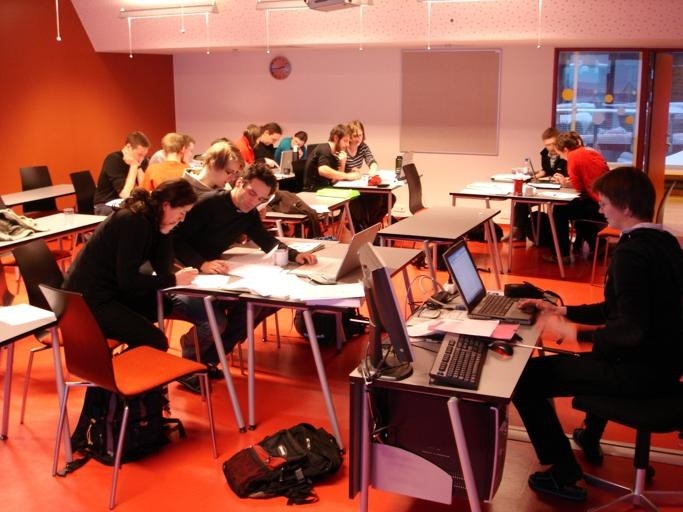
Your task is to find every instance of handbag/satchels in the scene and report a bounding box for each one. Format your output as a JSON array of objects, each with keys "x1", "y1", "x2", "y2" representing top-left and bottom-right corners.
[
  {"x1": 294, "y1": 310, "x2": 363, "y2": 346},
  {"x1": 222, "y1": 424, "x2": 341, "y2": 500},
  {"x1": 71, "y1": 386, "x2": 162, "y2": 465}
]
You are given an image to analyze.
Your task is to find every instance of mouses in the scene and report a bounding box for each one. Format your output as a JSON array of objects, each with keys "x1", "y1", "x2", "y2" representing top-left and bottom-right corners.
[{"x1": 488, "y1": 341, "x2": 512, "y2": 356}]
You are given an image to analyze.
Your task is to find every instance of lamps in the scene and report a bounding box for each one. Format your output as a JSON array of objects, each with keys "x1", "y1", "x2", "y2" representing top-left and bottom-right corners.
[
  {"x1": 252, "y1": 1, "x2": 377, "y2": 14},
  {"x1": 114, "y1": 4, "x2": 219, "y2": 21}
]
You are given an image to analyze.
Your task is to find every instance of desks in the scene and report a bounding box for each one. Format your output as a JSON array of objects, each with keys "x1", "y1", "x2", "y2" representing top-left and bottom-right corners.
[{"x1": 1, "y1": 157, "x2": 683, "y2": 511}]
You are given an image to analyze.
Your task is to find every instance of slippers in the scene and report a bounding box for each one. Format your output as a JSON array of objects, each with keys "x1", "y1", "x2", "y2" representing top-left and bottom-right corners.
[
  {"x1": 527, "y1": 471, "x2": 587, "y2": 500},
  {"x1": 572, "y1": 428, "x2": 603, "y2": 465}
]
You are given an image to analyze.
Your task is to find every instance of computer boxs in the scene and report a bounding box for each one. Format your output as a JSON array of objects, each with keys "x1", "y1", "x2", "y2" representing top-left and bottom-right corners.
[{"x1": 368, "y1": 385, "x2": 507, "y2": 503}]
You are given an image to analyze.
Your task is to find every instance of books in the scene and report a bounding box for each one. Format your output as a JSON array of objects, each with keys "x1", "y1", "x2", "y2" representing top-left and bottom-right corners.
[
  {"x1": 527, "y1": 182, "x2": 562, "y2": 190},
  {"x1": 490, "y1": 173, "x2": 531, "y2": 183}
]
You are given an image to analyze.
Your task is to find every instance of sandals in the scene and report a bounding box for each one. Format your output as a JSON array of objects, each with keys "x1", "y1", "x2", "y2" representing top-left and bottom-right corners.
[{"x1": 177, "y1": 364, "x2": 224, "y2": 394}]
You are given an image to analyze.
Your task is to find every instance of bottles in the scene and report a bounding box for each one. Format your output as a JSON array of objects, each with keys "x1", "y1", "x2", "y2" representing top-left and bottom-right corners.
[
  {"x1": 524, "y1": 158, "x2": 530, "y2": 175},
  {"x1": 292, "y1": 145, "x2": 298, "y2": 161},
  {"x1": 395, "y1": 155, "x2": 403, "y2": 175}
]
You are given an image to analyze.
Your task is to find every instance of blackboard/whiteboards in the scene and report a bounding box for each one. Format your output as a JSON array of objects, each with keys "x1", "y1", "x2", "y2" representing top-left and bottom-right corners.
[{"x1": 399, "y1": 48, "x2": 503, "y2": 157}]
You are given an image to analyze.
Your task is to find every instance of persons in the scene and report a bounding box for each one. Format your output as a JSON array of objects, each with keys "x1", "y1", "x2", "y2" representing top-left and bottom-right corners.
[
  {"x1": 540, "y1": 131, "x2": 610, "y2": 266},
  {"x1": 509, "y1": 127, "x2": 569, "y2": 245},
  {"x1": 510, "y1": 166, "x2": 683, "y2": 502}
]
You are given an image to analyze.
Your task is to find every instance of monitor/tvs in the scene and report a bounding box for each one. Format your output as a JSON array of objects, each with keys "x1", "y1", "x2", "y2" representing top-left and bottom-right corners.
[{"x1": 357, "y1": 241, "x2": 415, "y2": 381}]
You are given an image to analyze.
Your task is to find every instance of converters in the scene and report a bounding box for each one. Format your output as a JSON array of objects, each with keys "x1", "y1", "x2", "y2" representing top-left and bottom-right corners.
[{"x1": 427, "y1": 291, "x2": 448, "y2": 308}]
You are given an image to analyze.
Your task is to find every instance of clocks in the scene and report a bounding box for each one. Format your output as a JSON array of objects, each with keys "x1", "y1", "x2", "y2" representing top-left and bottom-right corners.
[{"x1": 269, "y1": 56, "x2": 292, "y2": 81}]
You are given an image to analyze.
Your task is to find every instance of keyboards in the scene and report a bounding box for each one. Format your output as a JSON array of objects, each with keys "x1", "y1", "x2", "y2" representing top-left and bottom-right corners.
[{"x1": 429, "y1": 332, "x2": 486, "y2": 389}]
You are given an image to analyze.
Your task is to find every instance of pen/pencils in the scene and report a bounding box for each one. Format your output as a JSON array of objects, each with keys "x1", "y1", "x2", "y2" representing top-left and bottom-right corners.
[
  {"x1": 174, "y1": 263, "x2": 185, "y2": 271},
  {"x1": 529, "y1": 160, "x2": 538, "y2": 182},
  {"x1": 494, "y1": 340, "x2": 543, "y2": 350}
]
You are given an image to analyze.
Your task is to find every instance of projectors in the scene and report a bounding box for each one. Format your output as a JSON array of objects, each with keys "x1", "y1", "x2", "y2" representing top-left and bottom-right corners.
[{"x1": 304, "y1": 0, "x2": 361, "y2": 11}]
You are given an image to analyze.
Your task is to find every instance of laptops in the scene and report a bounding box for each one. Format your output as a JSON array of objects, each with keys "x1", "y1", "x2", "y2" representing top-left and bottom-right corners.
[
  {"x1": 443, "y1": 240, "x2": 536, "y2": 325},
  {"x1": 289, "y1": 222, "x2": 381, "y2": 281},
  {"x1": 272, "y1": 151, "x2": 293, "y2": 172}
]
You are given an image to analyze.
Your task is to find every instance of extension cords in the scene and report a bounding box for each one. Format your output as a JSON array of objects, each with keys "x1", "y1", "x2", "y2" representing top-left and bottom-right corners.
[{"x1": 443, "y1": 281, "x2": 457, "y2": 294}]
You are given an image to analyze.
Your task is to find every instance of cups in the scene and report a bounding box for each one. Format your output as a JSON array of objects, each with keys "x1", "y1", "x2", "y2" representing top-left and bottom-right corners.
[
  {"x1": 275, "y1": 249, "x2": 289, "y2": 266},
  {"x1": 62, "y1": 207, "x2": 74, "y2": 222},
  {"x1": 512, "y1": 179, "x2": 524, "y2": 196}
]
